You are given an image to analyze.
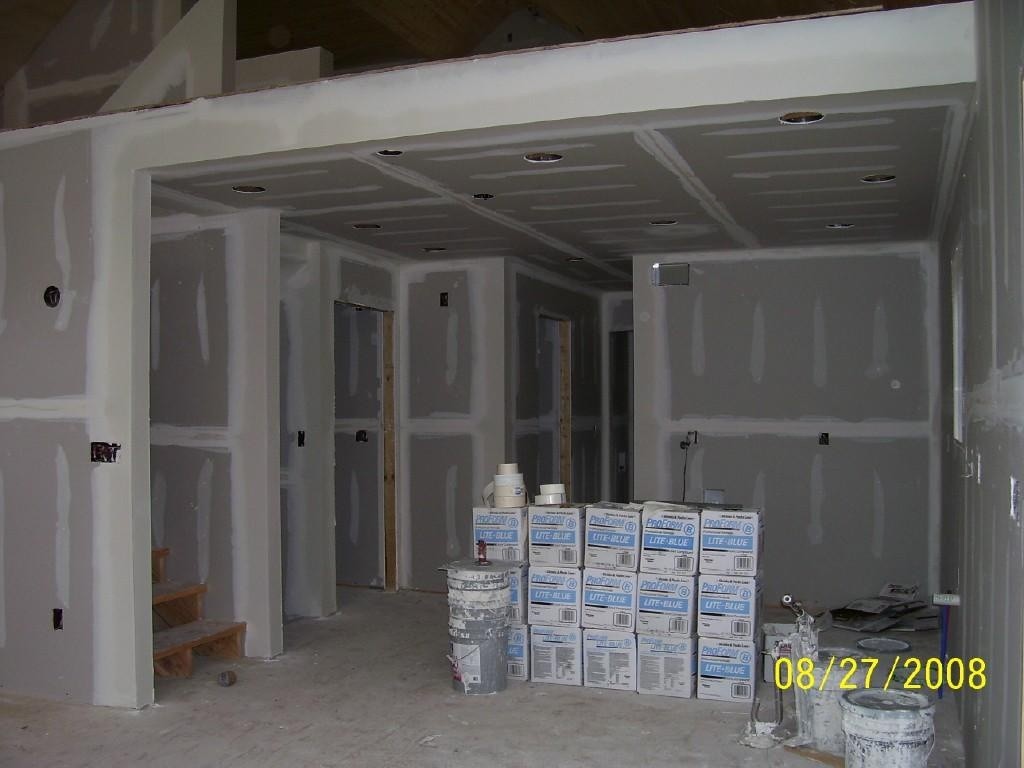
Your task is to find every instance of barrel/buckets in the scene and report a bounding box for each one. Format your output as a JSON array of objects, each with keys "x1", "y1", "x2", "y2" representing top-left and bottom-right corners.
[
  {"x1": 809, "y1": 647, "x2": 871, "y2": 752},
  {"x1": 447, "y1": 569, "x2": 511, "y2": 695},
  {"x1": 841, "y1": 687, "x2": 937, "y2": 768}
]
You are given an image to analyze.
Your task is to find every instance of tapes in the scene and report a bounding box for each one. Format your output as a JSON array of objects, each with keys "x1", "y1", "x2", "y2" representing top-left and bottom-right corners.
[
  {"x1": 494, "y1": 473, "x2": 526, "y2": 508},
  {"x1": 497, "y1": 463, "x2": 519, "y2": 474},
  {"x1": 539, "y1": 484, "x2": 566, "y2": 494},
  {"x1": 535, "y1": 494, "x2": 567, "y2": 505}
]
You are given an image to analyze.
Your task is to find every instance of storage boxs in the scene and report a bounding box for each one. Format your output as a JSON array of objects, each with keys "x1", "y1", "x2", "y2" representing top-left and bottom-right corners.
[
  {"x1": 530, "y1": 625, "x2": 583, "y2": 686},
  {"x1": 697, "y1": 576, "x2": 763, "y2": 637},
  {"x1": 504, "y1": 625, "x2": 530, "y2": 681},
  {"x1": 698, "y1": 637, "x2": 763, "y2": 704},
  {"x1": 637, "y1": 633, "x2": 697, "y2": 699},
  {"x1": 583, "y1": 500, "x2": 642, "y2": 571},
  {"x1": 636, "y1": 571, "x2": 698, "y2": 637},
  {"x1": 528, "y1": 503, "x2": 588, "y2": 567},
  {"x1": 581, "y1": 567, "x2": 637, "y2": 633},
  {"x1": 510, "y1": 562, "x2": 529, "y2": 624},
  {"x1": 640, "y1": 502, "x2": 701, "y2": 576},
  {"x1": 699, "y1": 504, "x2": 763, "y2": 577},
  {"x1": 583, "y1": 628, "x2": 636, "y2": 691},
  {"x1": 528, "y1": 566, "x2": 583, "y2": 627},
  {"x1": 473, "y1": 505, "x2": 528, "y2": 562},
  {"x1": 765, "y1": 623, "x2": 819, "y2": 684}
]
